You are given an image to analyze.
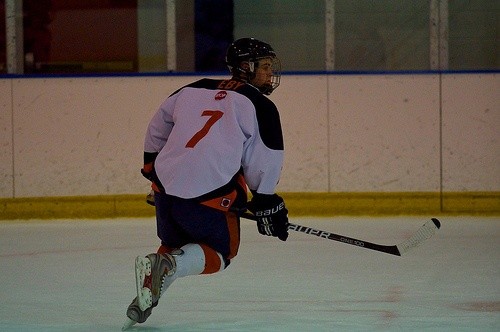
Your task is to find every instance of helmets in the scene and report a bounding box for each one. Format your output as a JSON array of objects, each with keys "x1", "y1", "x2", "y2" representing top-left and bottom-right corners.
[{"x1": 226, "y1": 38, "x2": 281, "y2": 95}]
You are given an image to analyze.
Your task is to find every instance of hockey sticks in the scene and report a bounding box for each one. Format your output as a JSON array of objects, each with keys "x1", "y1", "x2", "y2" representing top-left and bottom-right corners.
[{"x1": 146, "y1": 187, "x2": 440, "y2": 256}]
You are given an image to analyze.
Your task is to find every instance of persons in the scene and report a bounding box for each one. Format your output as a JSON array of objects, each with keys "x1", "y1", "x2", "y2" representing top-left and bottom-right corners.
[{"x1": 122, "y1": 37, "x2": 289, "y2": 332}]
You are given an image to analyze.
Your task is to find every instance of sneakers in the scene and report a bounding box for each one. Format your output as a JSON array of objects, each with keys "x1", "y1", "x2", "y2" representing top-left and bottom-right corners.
[
  {"x1": 122, "y1": 297, "x2": 158, "y2": 330},
  {"x1": 135, "y1": 253, "x2": 176, "y2": 312}
]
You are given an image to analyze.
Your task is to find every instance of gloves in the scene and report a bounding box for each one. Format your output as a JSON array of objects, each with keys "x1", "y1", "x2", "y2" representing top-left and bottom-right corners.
[{"x1": 247, "y1": 194, "x2": 289, "y2": 241}]
